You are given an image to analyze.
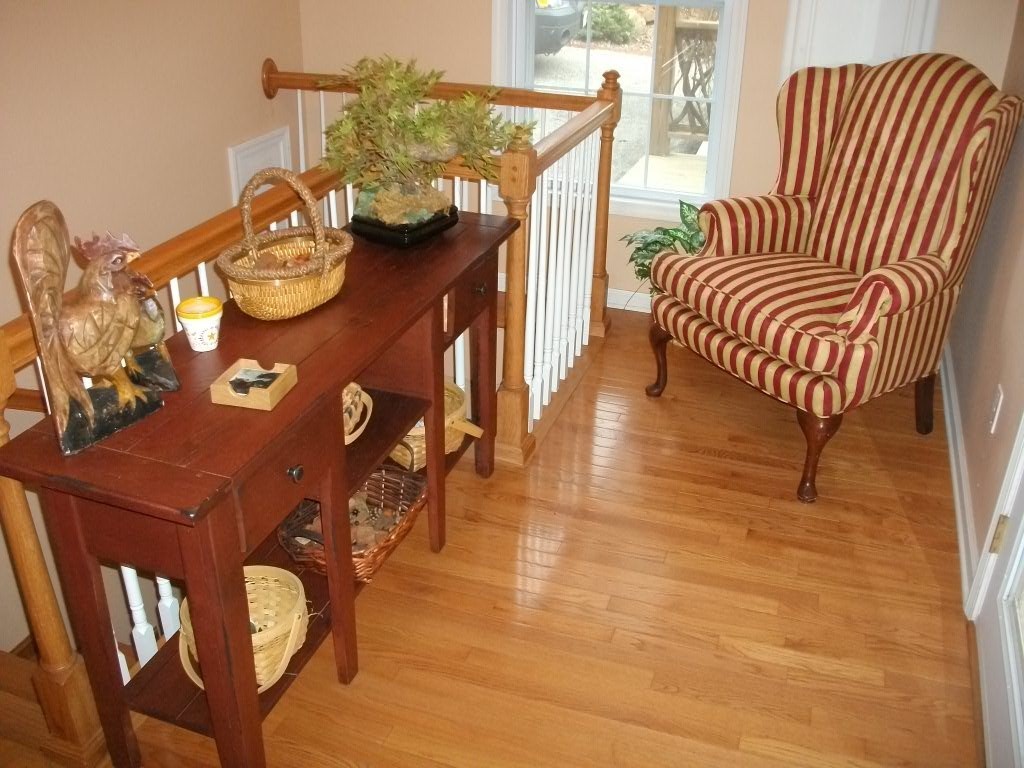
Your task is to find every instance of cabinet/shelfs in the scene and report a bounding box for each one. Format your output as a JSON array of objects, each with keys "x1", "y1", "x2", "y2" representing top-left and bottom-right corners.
[{"x1": 0, "y1": 208, "x2": 521, "y2": 768}]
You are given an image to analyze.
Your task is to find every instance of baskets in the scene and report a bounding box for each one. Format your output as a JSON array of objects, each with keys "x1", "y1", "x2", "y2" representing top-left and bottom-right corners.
[
  {"x1": 175, "y1": 570, "x2": 308, "y2": 694},
  {"x1": 209, "y1": 166, "x2": 356, "y2": 320},
  {"x1": 277, "y1": 440, "x2": 430, "y2": 583},
  {"x1": 390, "y1": 380, "x2": 485, "y2": 469}
]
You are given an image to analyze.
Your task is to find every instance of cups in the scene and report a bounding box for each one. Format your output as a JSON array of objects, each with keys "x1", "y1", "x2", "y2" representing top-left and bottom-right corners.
[{"x1": 176, "y1": 296, "x2": 223, "y2": 352}]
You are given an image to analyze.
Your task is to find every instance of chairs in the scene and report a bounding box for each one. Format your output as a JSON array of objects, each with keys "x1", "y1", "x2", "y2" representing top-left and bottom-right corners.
[{"x1": 645, "y1": 52, "x2": 1024, "y2": 504}]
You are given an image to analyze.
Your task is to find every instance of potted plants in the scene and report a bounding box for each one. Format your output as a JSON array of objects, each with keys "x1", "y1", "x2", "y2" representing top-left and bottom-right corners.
[
  {"x1": 316, "y1": 50, "x2": 540, "y2": 248},
  {"x1": 618, "y1": 200, "x2": 706, "y2": 347}
]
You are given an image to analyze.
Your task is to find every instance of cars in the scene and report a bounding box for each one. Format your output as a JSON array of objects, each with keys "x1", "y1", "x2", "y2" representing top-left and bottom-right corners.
[{"x1": 535, "y1": 0, "x2": 587, "y2": 56}]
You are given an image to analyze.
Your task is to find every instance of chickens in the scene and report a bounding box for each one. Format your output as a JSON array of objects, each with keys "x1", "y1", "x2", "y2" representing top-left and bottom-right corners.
[{"x1": 10, "y1": 202, "x2": 153, "y2": 436}]
